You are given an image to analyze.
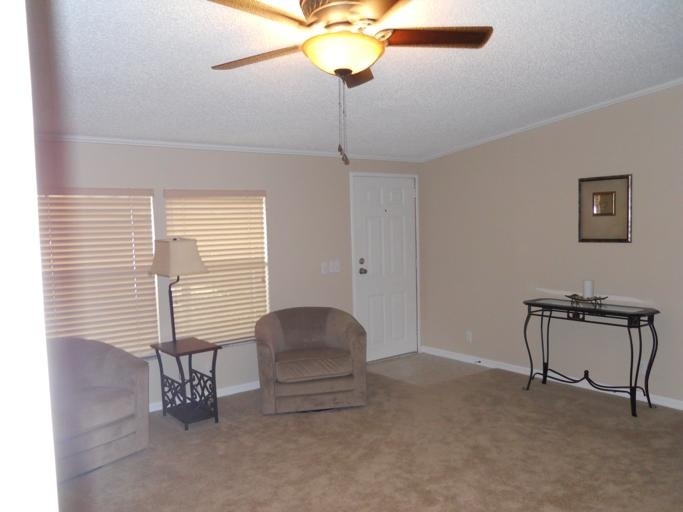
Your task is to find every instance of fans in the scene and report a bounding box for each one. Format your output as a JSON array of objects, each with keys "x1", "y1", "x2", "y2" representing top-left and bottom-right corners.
[{"x1": 206, "y1": 1, "x2": 494, "y2": 90}]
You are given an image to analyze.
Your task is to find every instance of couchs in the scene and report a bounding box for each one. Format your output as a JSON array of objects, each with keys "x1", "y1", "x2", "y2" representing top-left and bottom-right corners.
[
  {"x1": 254, "y1": 306, "x2": 369, "y2": 416},
  {"x1": 45, "y1": 337, "x2": 149, "y2": 485}
]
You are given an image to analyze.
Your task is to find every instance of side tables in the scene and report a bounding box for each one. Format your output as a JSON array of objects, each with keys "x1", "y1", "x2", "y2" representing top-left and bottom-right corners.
[{"x1": 150, "y1": 336, "x2": 223, "y2": 427}]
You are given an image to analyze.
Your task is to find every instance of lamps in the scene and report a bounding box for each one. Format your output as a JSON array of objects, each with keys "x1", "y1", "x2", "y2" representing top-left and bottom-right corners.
[
  {"x1": 294, "y1": 20, "x2": 398, "y2": 81},
  {"x1": 147, "y1": 238, "x2": 208, "y2": 341}
]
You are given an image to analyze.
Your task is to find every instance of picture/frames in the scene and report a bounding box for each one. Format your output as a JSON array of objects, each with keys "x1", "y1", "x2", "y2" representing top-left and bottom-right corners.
[{"x1": 575, "y1": 174, "x2": 630, "y2": 244}]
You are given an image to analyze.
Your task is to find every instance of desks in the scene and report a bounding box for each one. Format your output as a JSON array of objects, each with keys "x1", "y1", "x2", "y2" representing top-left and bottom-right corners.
[{"x1": 524, "y1": 296, "x2": 658, "y2": 419}]
